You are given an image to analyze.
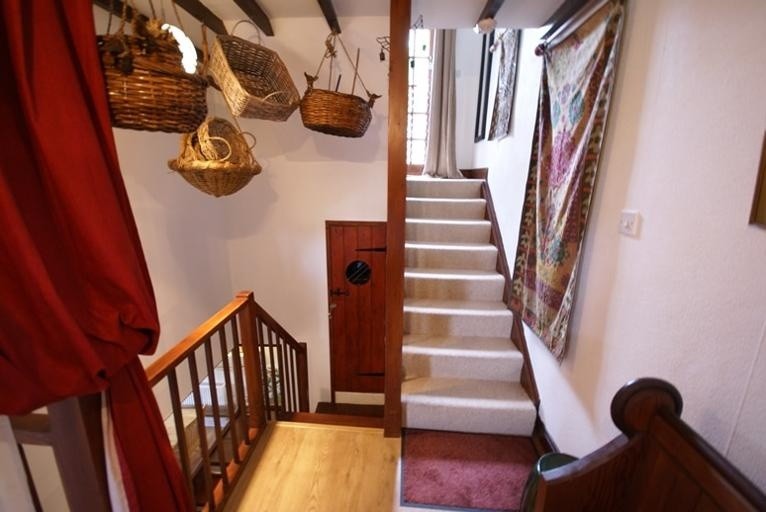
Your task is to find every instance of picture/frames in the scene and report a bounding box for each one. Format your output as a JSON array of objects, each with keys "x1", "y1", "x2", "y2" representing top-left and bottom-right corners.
[{"x1": 474, "y1": 29, "x2": 495, "y2": 143}]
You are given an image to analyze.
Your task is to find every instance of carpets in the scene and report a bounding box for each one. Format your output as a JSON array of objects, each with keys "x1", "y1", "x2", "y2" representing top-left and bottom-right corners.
[{"x1": 402, "y1": 426, "x2": 542, "y2": 511}]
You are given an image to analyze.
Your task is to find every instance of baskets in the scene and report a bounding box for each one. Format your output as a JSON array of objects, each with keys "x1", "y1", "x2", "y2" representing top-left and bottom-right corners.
[
  {"x1": 96, "y1": 1, "x2": 183, "y2": 69},
  {"x1": 301, "y1": 31, "x2": 382, "y2": 137},
  {"x1": 101, "y1": 3, "x2": 208, "y2": 132},
  {"x1": 168, "y1": 117, "x2": 261, "y2": 197},
  {"x1": 210, "y1": 19, "x2": 302, "y2": 121}
]
